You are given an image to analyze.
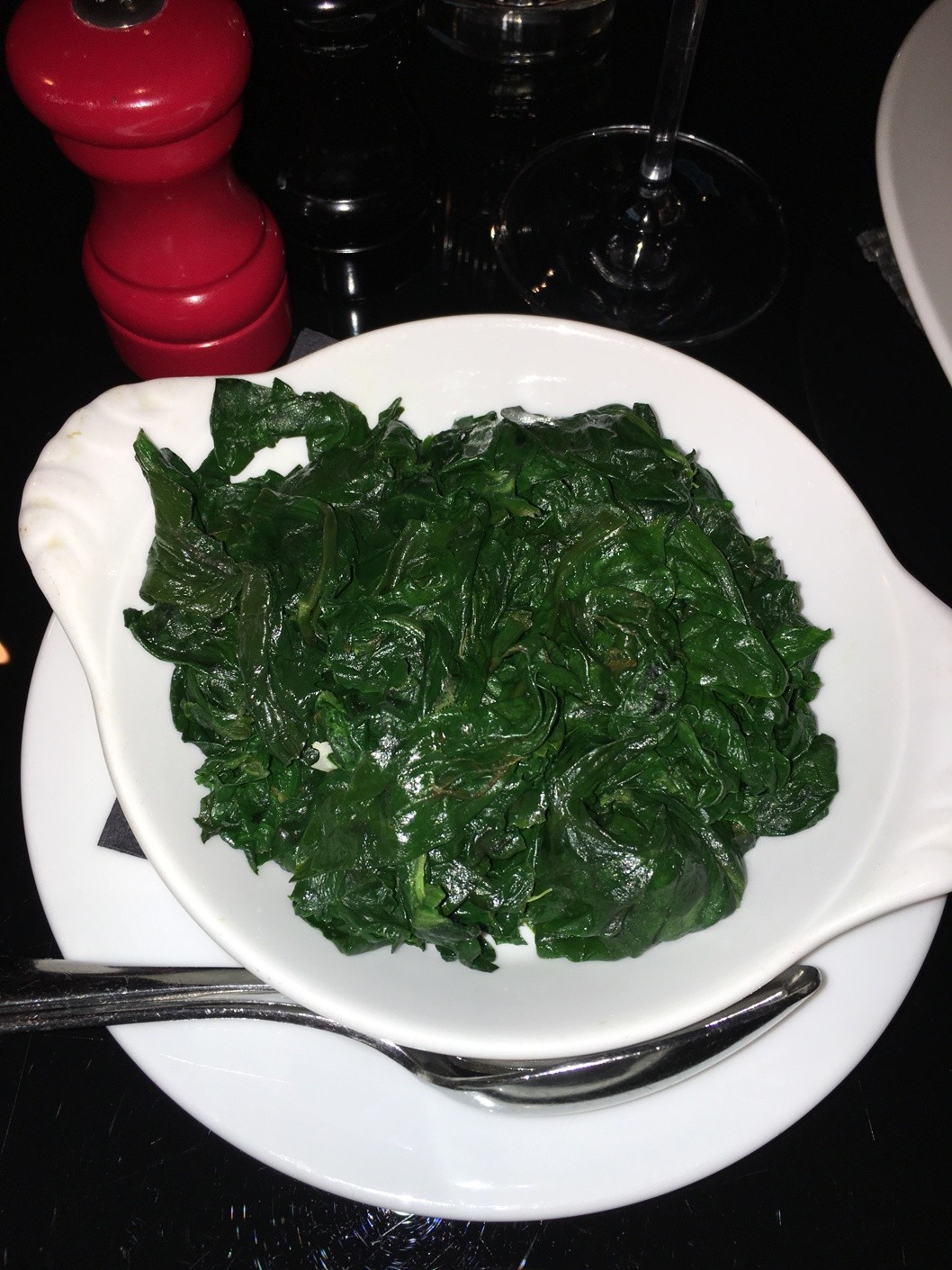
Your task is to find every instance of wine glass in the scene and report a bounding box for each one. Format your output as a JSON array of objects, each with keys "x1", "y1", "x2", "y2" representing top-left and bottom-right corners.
[{"x1": 487, "y1": 0, "x2": 790, "y2": 348}]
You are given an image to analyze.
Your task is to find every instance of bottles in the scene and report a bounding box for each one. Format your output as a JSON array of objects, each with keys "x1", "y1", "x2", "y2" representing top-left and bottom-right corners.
[{"x1": 6, "y1": 1, "x2": 289, "y2": 381}]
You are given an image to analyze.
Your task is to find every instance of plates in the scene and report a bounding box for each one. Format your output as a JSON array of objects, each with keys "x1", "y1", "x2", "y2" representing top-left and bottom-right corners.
[
  {"x1": 874, "y1": 0, "x2": 952, "y2": 390},
  {"x1": 21, "y1": 609, "x2": 945, "y2": 1222},
  {"x1": 18, "y1": 312, "x2": 952, "y2": 1063}
]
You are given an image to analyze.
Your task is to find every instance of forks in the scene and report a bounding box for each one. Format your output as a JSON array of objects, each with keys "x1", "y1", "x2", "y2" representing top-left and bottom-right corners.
[{"x1": 0, "y1": 988, "x2": 788, "y2": 1089}]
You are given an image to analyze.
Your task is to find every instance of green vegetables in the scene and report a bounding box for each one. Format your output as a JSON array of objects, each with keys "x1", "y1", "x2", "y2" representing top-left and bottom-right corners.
[{"x1": 123, "y1": 370, "x2": 840, "y2": 974}]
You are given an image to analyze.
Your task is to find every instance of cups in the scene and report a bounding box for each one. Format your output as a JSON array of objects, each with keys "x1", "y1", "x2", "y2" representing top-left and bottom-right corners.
[{"x1": 260, "y1": 1, "x2": 618, "y2": 317}]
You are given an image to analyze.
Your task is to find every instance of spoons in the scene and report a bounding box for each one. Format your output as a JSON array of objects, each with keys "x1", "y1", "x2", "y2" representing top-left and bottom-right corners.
[{"x1": 1, "y1": 959, "x2": 821, "y2": 1107}]
